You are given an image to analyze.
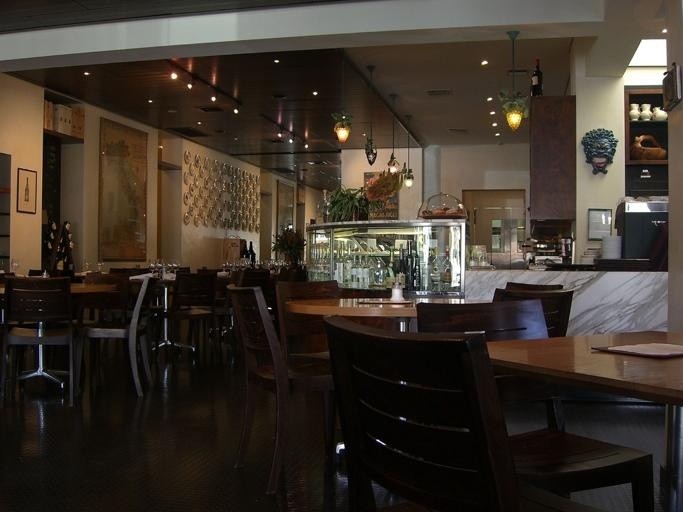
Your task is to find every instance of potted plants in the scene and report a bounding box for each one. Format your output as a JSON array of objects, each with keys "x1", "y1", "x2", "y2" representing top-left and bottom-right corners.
[{"x1": 324, "y1": 183, "x2": 369, "y2": 222}]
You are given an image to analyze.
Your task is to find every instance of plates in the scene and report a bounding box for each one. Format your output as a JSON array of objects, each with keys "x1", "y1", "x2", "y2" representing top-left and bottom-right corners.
[{"x1": 182, "y1": 149, "x2": 262, "y2": 233}]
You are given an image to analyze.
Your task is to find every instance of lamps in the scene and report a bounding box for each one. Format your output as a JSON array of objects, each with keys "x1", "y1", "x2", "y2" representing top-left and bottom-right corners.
[
  {"x1": 388, "y1": 93, "x2": 400, "y2": 173},
  {"x1": 404, "y1": 114, "x2": 415, "y2": 187},
  {"x1": 330, "y1": 54, "x2": 354, "y2": 142},
  {"x1": 498, "y1": 30, "x2": 531, "y2": 130},
  {"x1": 365, "y1": 64, "x2": 378, "y2": 165}
]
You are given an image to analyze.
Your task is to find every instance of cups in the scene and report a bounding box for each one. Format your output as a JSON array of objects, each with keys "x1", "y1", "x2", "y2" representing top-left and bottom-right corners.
[
  {"x1": 11, "y1": 258, "x2": 20, "y2": 273},
  {"x1": 79, "y1": 255, "x2": 104, "y2": 272}
]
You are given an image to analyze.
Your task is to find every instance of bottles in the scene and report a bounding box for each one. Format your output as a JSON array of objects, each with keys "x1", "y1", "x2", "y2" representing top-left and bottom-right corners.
[
  {"x1": 241, "y1": 243, "x2": 248, "y2": 262},
  {"x1": 248, "y1": 240, "x2": 256, "y2": 267},
  {"x1": 468, "y1": 245, "x2": 488, "y2": 267},
  {"x1": 0, "y1": 258, "x2": 6, "y2": 274},
  {"x1": 308, "y1": 239, "x2": 453, "y2": 300},
  {"x1": 23, "y1": 176, "x2": 30, "y2": 202}
]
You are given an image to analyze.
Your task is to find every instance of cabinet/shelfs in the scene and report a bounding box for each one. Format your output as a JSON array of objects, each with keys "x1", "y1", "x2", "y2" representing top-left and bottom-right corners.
[
  {"x1": 306, "y1": 218, "x2": 466, "y2": 300},
  {"x1": 624, "y1": 85, "x2": 670, "y2": 196}
]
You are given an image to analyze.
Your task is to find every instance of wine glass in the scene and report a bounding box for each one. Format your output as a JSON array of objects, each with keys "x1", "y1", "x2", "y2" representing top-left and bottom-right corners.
[
  {"x1": 147, "y1": 258, "x2": 179, "y2": 280},
  {"x1": 219, "y1": 260, "x2": 287, "y2": 275}
]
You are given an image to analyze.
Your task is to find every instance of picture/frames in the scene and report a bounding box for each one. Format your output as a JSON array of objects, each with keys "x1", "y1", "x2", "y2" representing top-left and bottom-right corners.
[
  {"x1": 588, "y1": 207, "x2": 613, "y2": 241},
  {"x1": 16, "y1": 167, "x2": 37, "y2": 215},
  {"x1": 97, "y1": 115, "x2": 149, "y2": 262}
]
